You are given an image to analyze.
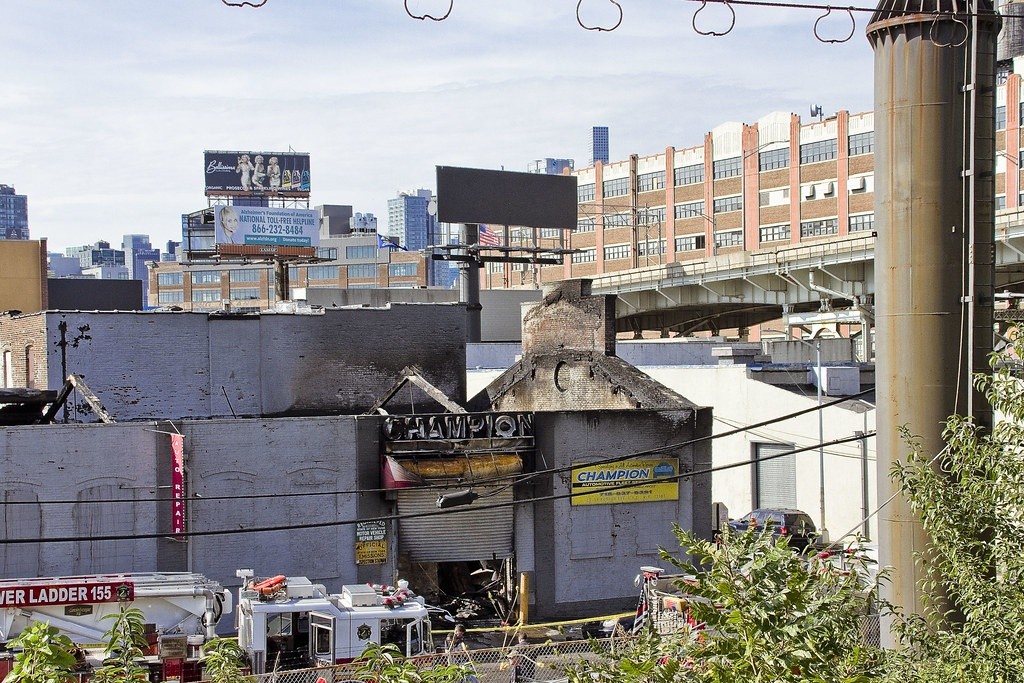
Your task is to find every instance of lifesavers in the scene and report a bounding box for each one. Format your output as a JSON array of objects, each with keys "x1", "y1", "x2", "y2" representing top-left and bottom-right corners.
[
  {"x1": 249, "y1": 577, "x2": 281, "y2": 593},
  {"x1": 255, "y1": 573, "x2": 285, "y2": 589}
]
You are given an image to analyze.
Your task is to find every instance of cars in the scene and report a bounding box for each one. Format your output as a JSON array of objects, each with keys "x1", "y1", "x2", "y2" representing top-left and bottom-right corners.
[{"x1": 728, "y1": 504, "x2": 816, "y2": 553}]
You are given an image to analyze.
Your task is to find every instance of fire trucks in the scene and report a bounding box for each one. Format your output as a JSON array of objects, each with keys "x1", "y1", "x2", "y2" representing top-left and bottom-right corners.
[{"x1": 0, "y1": 570, "x2": 456, "y2": 683}]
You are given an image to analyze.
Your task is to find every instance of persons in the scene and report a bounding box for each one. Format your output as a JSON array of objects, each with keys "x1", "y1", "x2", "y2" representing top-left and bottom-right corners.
[
  {"x1": 236, "y1": 155, "x2": 280, "y2": 191},
  {"x1": 509, "y1": 632, "x2": 538, "y2": 683},
  {"x1": 445, "y1": 624, "x2": 468, "y2": 666},
  {"x1": 220, "y1": 206, "x2": 238, "y2": 243}
]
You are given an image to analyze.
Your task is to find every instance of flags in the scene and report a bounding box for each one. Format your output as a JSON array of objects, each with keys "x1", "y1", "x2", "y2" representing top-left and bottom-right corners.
[
  {"x1": 633, "y1": 587, "x2": 647, "y2": 636},
  {"x1": 478, "y1": 223, "x2": 500, "y2": 245},
  {"x1": 378, "y1": 233, "x2": 408, "y2": 250}
]
